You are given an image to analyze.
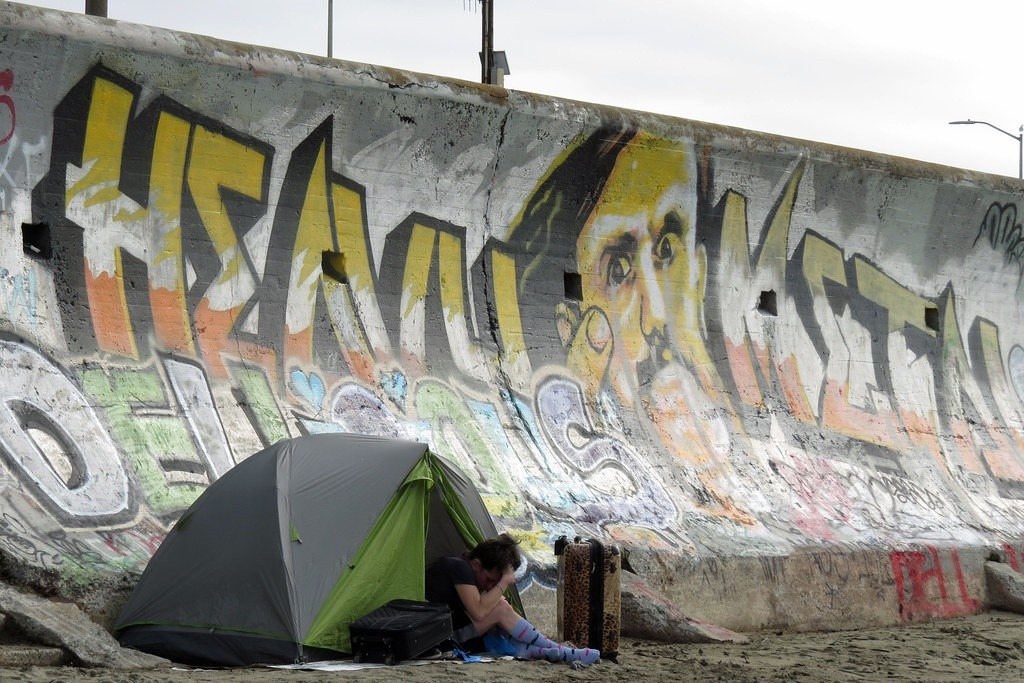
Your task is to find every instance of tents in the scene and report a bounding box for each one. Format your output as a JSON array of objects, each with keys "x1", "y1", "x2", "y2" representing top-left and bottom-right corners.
[{"x1": 111, "y1": 431, "x2": 531, "y2": 665}]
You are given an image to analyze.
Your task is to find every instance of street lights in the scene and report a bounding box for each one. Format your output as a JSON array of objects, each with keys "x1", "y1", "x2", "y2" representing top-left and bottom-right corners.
[{"x1": 948, "y1": 120, "x2": 1023, "y2": 178}]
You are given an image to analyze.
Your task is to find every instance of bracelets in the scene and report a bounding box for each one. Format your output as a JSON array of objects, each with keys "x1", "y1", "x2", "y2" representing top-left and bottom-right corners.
[{"x1": 496, "y1": 584, "x2": 505, "y2": 596}]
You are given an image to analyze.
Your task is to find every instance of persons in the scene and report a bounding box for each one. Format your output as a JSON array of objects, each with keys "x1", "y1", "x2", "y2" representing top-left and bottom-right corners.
[{"x1": 425, "y1": 533, "x2": 600, "y2": 665}]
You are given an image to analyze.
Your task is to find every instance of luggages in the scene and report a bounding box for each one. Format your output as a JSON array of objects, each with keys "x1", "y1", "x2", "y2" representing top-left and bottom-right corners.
[
  {"x1": 557, "y1": 540, "x2": 620, "y2": 657},
  {"x1": 349, "y1": 598, "x2": 456, "y2": 666}
]
